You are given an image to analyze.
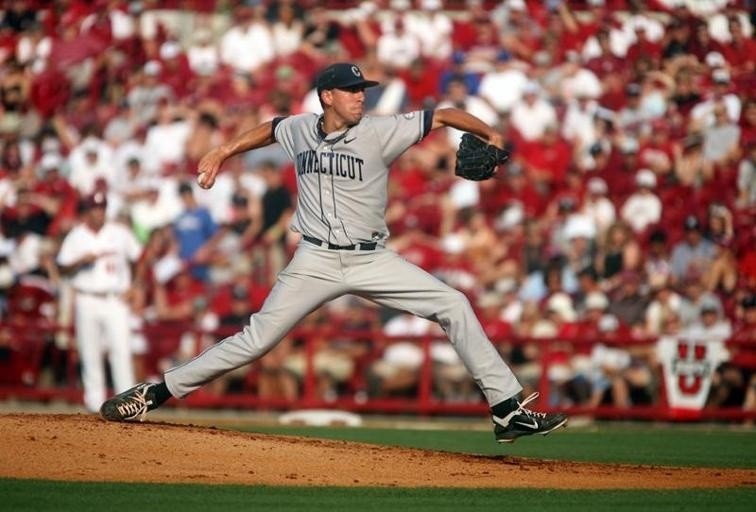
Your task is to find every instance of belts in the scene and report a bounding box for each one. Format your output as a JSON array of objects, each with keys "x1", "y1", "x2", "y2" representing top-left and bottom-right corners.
[{"x1": 303, "y1": 234, "x2": 378, "y2": 250}]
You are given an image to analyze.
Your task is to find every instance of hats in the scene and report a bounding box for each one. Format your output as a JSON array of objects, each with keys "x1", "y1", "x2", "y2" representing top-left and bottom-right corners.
[{"x1": 316, "y1": 64, "x2": 380, "y2": 97}]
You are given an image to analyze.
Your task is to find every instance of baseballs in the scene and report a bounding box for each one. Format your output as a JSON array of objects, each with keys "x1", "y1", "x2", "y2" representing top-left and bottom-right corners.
[{"x1": 196, "y1": 173, "x2": 214, "y2": 189}]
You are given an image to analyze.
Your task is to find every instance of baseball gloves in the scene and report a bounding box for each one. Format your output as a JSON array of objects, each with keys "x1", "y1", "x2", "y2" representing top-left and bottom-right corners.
[{"x1": 455, "y1": 133, "x2": 510, "y2": 181}]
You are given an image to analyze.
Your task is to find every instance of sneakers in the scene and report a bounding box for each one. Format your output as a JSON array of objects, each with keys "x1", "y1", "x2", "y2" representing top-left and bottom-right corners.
[
  {"x1": 99, "y1": 382, "x2": 158, "y2": 422},
  {"x1": 492, "y1": 391, "x2": 569, "y2": 444}
]
